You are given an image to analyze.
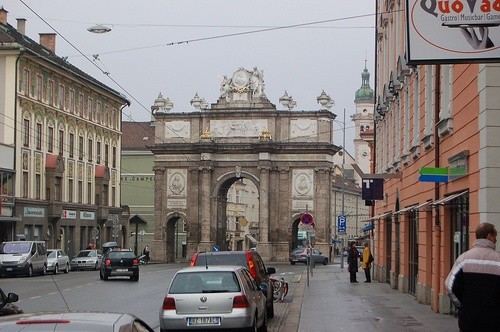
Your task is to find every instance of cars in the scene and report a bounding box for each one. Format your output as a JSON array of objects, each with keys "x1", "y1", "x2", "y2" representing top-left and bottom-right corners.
[
  {"x1": 46, "y1": 249, "x2": 71, "y2": 275},
  {"x1": 70, "y1": 249, "x2": 104, "y2": 270},
  {"x1": 289, "y1": 247, "x2": 328, "y2": 266},
  {"x1": 0, "y1": 312, "x2": 156, "y2": 332},
  {"x1": 264, "y1": 265, "x2": 288, "y2": 302},
  {"x1": 0, "y1": 286, "x2": 24, "y2": 317},
  {"x1": 159, "y1": 265, "x2": 269, "y2": 332}
]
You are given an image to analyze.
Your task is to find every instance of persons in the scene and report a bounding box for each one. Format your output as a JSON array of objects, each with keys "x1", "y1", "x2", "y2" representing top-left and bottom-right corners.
[
  {"x1": 362, "y1": 243, "x2": 372, "y2": 283},
  {"x1": 251, "y1": 66, "x2": 259, "y2": 90},
  {"x1": 86, "y1": 244, "x2": 92, "y2": 250},
  {"x1": 221, "y1": 75, "x2": 228, "y2": 94},
  {"x1": 445, "y1": 222, "x2": 500, "y2": 332},
  {"x1": 347, "y1": 241, "x2": 359, "y2": 283}
]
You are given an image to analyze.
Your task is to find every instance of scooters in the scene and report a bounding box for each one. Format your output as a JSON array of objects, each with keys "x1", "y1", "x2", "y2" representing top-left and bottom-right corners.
[{"x1": 137, "y1": 255, "x2": 147, "y2": 265}]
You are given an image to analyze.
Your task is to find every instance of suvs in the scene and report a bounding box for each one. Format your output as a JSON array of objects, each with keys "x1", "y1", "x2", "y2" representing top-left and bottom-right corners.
[
  {"x1": 100, "y1": 248, "x2": 139, "y2": 281},
  {"x1": 189, "y1": 250, "x2": 274, "y2": 318},
  {"x1": 0, "y1": 241, "x2": 48, "y2": 277}
]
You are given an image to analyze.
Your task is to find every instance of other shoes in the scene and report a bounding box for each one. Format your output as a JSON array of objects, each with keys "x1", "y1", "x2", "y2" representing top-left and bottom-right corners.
[
  {"x1": 352, "y1": 280, "x2": 358, "y2": 283},
  {"x1": 364, "y1": 281, "x2": 370, "y2": 283}
]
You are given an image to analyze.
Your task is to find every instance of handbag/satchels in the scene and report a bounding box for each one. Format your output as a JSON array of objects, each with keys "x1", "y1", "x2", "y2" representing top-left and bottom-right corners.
[{"x1": 369, "y1": 252, "x2": 374, "y2": 262}]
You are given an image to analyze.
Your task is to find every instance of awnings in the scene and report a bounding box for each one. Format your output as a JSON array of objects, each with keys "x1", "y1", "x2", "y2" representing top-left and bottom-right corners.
[
  {"x1": 332, "y1": 239, "x2": 341, "y2": 243},
  {"x1": 246, "y1": 235, "x2": 258, "y2": 244},
  {"x1": 363, "y1": 226, "x2": 374, "y2": 232}
]
